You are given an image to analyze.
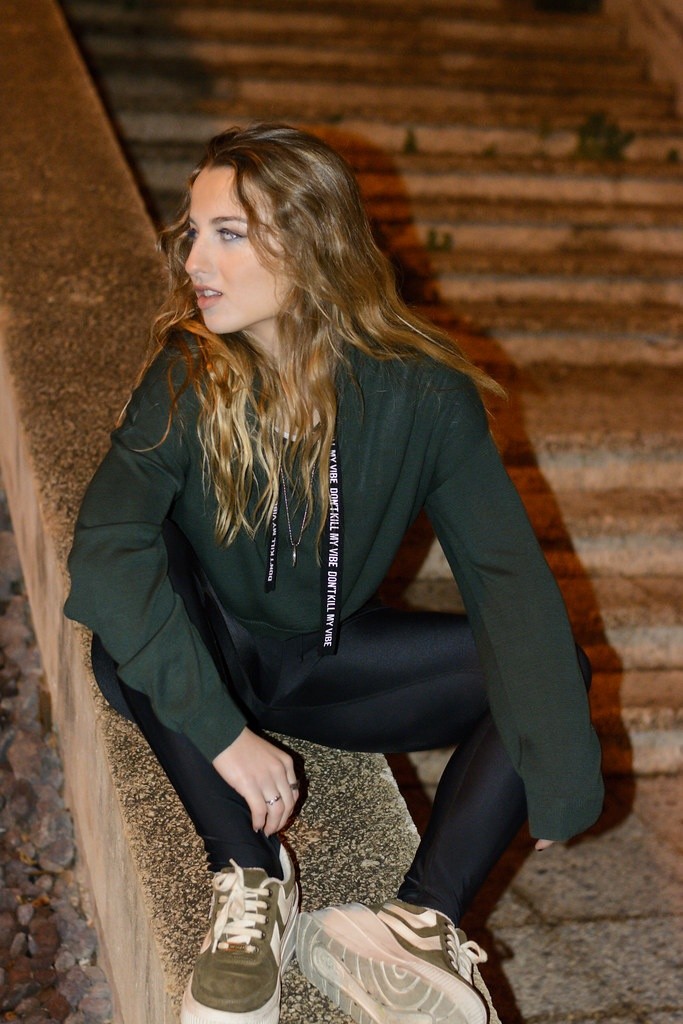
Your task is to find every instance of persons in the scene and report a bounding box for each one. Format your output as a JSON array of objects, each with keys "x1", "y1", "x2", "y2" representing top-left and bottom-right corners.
[{"x1": 61, "y1": 117, "x2": 607, "y2": 1024}]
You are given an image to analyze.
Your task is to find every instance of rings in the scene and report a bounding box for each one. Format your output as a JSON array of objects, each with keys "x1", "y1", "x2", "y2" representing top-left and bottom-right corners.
[
  {"x1": 289, "y1": 781, "x2": 301, "y2": 791},
  {"x1": 264, "y1": 792, "x2": 282, "y2": 805}
]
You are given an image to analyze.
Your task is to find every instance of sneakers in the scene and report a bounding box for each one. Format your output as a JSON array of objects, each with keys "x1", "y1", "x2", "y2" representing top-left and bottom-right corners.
[
  {"x1": 294, "y1": 897, "x2": 487, "y2": 1024},
  {"x1": 180, "y1": 837, "x2": 299, "y2": 1024}
]
